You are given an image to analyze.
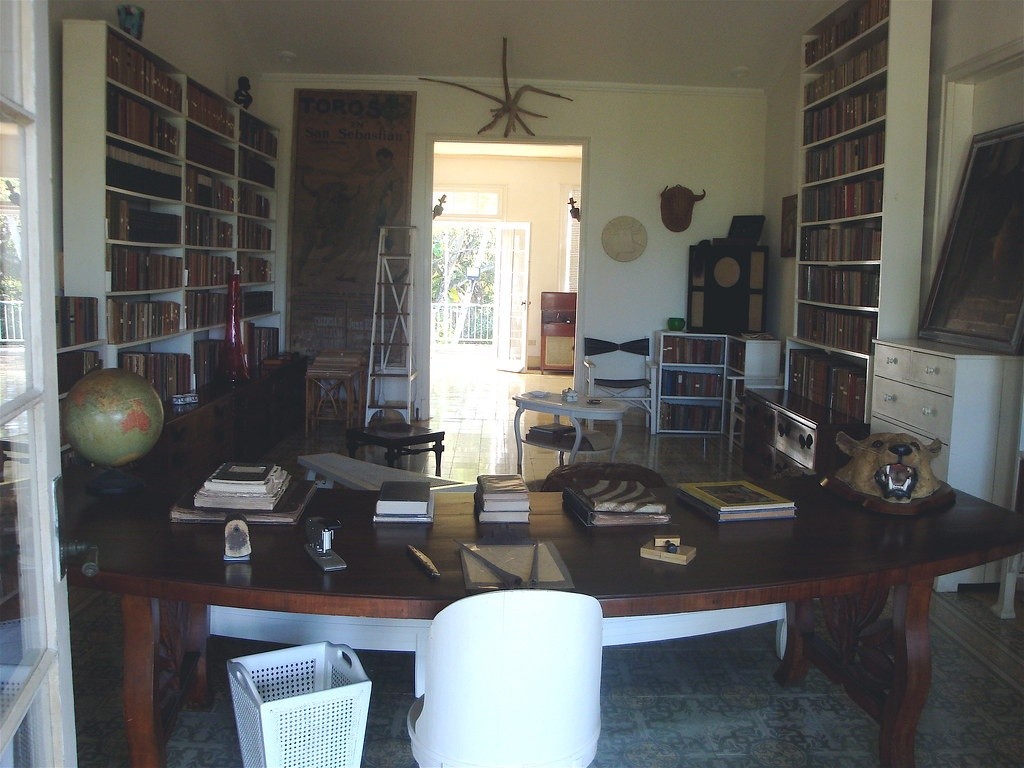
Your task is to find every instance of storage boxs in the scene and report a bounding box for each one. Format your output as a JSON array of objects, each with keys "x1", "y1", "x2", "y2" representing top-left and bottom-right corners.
[{"x1": 226, "y1": 642, "x2": 372, "y2": 768}]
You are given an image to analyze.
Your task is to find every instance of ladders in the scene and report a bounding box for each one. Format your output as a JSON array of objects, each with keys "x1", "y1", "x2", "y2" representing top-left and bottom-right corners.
[{"x1": 365, "y1": 226, "x2": 417, "y2": 428}]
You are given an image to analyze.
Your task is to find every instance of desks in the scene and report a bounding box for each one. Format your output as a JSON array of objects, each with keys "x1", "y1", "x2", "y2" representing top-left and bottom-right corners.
[
  {"x1": 62, "y1": 471, "x2": 1023, "y2": 768},
  {"x1": 512, "y1": 391, "x2": 629, "y2": 478}
]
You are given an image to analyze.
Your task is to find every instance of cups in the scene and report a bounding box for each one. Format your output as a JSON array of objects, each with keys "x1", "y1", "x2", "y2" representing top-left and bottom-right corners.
[{"x1": 118, "y1": 5, "x2": 145, "y2": 41}]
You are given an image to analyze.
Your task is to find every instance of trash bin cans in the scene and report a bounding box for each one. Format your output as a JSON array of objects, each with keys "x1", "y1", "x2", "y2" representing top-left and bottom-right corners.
[{"x1": 226, "y1": 641, "x2": 372, "y2": 768}]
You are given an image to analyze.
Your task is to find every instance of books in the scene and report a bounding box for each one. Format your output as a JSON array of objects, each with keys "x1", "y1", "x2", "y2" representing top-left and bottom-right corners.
[
  {"x1": 788, "y1": 0, "x2": 889, "y2": 421},
  {"x1": 55, "y1": 294, "x2": 98, "y2": 394},
  {"x1": 169, "y1": 462, "x2": 319, "y2": 526},
  {"x1": 474, "y1": 475, "x2": 532, "y2": 524},
  {"x1": 659, "y1": 336, "x2": 745, "y2": 441},
  {"x1": 373, "y1": 481, "x2": 435, "y2": 523},
  {"x1": 106, "y1": 31, "x2": 279, "y2": 402},
  {"x1": 561, "y1": 479, "x2": 672, "y2": 526},
  {"x1": 534, "y1": 422, "x2": 574, "y2": 434},
  {"x1": 675, "y1": 480, "x2": 798, "y2": 521}
]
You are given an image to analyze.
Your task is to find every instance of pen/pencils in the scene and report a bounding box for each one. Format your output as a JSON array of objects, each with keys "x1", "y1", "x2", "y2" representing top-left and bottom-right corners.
[{"x1": 537, "y1": 399, "x2": 562, "y2": 406}]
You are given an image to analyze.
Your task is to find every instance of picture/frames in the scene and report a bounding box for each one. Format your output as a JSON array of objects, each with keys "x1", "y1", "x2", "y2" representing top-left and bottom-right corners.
[{"x1": 919, "y1": 123, "x2": 1023, "y2": 355}]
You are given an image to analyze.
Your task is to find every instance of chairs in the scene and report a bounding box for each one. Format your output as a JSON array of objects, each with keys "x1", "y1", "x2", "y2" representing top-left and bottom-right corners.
[
  {"x1": 584, "y1": 333, "x2": 657, "y2": 434},
  {"x1": 410, "y1": 588, "x2": 602, "y2": 768}
]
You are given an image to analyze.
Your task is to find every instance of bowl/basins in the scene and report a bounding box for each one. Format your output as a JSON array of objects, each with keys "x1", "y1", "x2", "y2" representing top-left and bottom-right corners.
[{"x1": 668, "y1": 318, "x2": 685, "y2": 331}]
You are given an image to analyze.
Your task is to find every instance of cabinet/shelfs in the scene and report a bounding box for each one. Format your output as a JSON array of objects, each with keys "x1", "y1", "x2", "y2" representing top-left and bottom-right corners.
[
  {"x1": 650, "y1": 0, "x2": 1024, "y2": 593},
  {"x1": 63, "y1": 19, "x2": 278, "y2": 392}
]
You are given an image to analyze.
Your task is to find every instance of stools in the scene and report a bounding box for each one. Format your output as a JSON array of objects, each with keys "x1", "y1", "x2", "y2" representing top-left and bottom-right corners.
[
  {"x1": 303, "y1": 348, "x2": 365, "y2": 436},
  {"x1": 347, "y1": 423, "x2": 447, "y2": 480}
]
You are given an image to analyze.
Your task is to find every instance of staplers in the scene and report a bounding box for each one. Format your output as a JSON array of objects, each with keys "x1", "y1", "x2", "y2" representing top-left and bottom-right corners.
[{"x1": 303, "y1": 516, "x2": 347, "y2": 572}]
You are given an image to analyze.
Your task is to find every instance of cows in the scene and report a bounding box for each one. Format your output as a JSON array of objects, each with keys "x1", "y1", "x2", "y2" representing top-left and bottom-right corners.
[{"x1": 661, "y1": 185, "x2": 706, "y2": 219}]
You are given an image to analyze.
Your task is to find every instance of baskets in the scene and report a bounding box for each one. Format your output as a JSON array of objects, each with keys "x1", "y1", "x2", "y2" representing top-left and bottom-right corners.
[{"x1": 226, "y1": 640, "x2": 372, "y2": 768}]
